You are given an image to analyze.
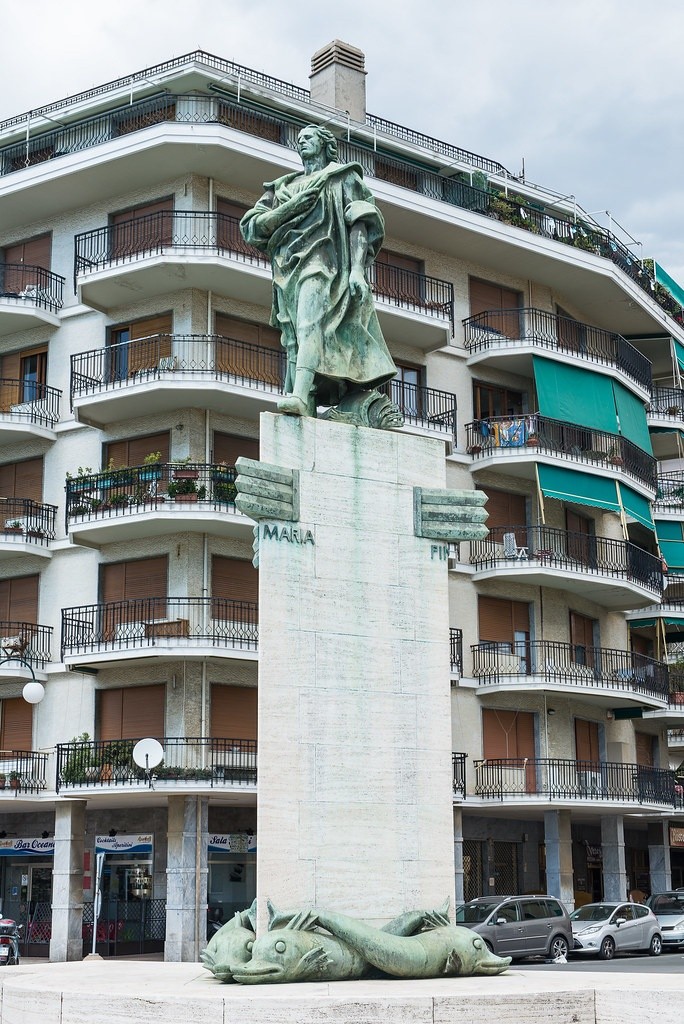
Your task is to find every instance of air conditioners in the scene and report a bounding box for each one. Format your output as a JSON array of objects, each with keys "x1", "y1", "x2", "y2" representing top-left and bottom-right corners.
[{"x1": 575, "y1": 771, "x2": 602, "y2": 795}]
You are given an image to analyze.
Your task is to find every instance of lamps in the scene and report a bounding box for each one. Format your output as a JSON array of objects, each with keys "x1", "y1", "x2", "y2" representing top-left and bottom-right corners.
[
  {"x1": 238, "y1": 828, "x2": 254, "y2": 836},
  {"x1": 548, "y1": 708, "x2": 556, "y2": 716},
  {"x1": 0, "y1": 830, "x2": 17, "y2": 838},
  {"x1": 41, "y1": 831, "x2": 55, "y2": 839},
  {"x1": 108, "y1": 828, "x2": 126, "y2": 836},
  {"x1": 176, "y1": 424, "x2": 184, "y2": 431},
  {"x1": 523, "y1": 832, "x2": 529, "y2": 842}
]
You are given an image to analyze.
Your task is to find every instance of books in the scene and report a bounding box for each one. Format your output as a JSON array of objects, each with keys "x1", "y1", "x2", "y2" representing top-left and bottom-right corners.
[{"x1": 319, "y1": 388, "x2": 405, "y2": 429}]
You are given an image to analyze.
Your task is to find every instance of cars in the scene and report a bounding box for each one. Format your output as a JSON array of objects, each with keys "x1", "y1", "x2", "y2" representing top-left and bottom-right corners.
[{"x1": 567, "y1": 902, "x2": 663, "y2": 960}]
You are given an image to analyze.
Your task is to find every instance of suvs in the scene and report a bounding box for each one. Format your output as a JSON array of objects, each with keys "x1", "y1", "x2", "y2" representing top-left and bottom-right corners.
[
  {"x1": 455, "y1": 894, "x2": 574, "y2": 962},
  {"x1": 638, "y1": 889, "x2": 684, "y2": 952}
]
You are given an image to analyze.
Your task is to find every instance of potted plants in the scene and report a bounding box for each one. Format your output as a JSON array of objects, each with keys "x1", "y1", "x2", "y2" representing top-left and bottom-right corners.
[
  {"x1": 526, "y1": 432, "x2": 541, "y2": 447},
  {"x1": 214, "y1": 481, "x2": 237, "y2": 503},
  {"x1": 167, "y1": 478, "x2": 207, "y2": 503},
  {"x1": 29, "y1": 526, "x2": 44, "y2": 538},
  {"x1": 144, "y1": 480, "x2": 165, "y2": 504},
  {"x1": 9, "y1": 771, "x2": 23, "y2": 789},
  {"x1": 63, "y1": 467, "x2": 95, "y2": 494},
  {"x1": 665, "y1": 405, "x2": 683, "y2": 416},
  {"x1": 97, "y1": 458, "x2": 132, "y2": 490},
  {"x1": 90, "y1": 497, "x2": 111, "y2": 512},
  {"x1": 0, "y1": 772, "x2": 6, "y2": 789},
  {"x1": 668, "y1": 657, "x2": 684, "y2": 705},
  {"x1": 110, "y1": 493, "x2": 129, "y2": 508},
  {"x1": 607, "y1": 439, "x2": 623, "y2": 465},
  {"x1": 210, "y1": 461, "x2": 236, "y2": 482},
  {"x1": 58, "y1": 732, "x2": 211, "y2": 784},
  {"x1": 138, "y1": 450, "x2": 165, "y2": 481},
  {"x1": 5, "y1": 521, "x2": 25, "y2": 533},
  {"x1": 170, "y1": 456, "x2": 199, "y2": 479}
]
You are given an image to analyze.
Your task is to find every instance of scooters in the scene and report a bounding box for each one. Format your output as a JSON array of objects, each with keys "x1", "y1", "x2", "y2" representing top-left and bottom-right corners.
[{"x1": 0, "y1": 914, "x2": 24, "y2": 966}]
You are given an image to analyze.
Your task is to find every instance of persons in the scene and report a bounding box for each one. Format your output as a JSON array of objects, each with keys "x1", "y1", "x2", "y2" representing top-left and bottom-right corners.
[{"x1": 237, "y1": 124, "x2": 399, "y2": 417}]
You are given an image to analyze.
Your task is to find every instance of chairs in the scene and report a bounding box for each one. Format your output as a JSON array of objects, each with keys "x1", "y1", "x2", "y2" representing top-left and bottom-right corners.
[
  {"x1": 129, "y1": 356, "x2": 177, "y2": 378},
  {"x1": 2, "y1": 630, "x2": 31, "y2": 666},
  {"x1": 502, "y1": 532, "x2": 530, "y2": 561},
  {"x1": 22, "y1": 283, "x2": 40, "y2": 300}
]
